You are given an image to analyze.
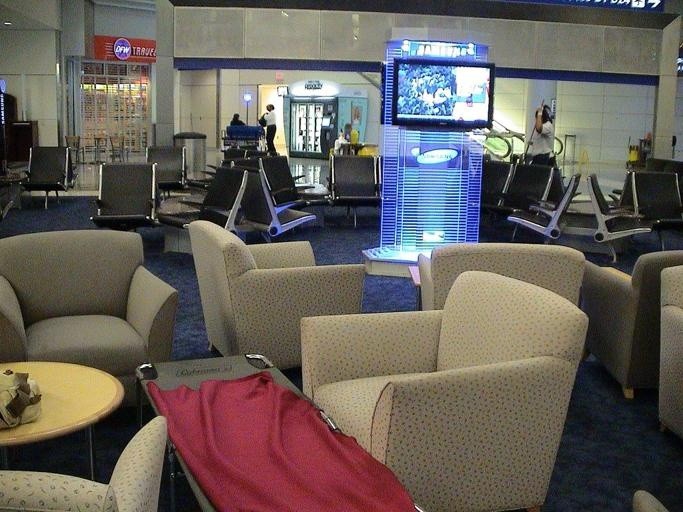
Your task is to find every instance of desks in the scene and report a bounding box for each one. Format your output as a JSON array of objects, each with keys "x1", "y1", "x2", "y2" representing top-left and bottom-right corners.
[
  {"x1": 0, "y1": 362, "x2": 125, "y2": 482},
  {"x1": 137, "y1": 353, "x2": 419, "y2": 508}
]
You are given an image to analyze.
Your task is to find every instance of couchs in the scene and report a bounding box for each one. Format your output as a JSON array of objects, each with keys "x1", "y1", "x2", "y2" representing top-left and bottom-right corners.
[
  {"x1": 419, "y1": 243, "x2": 587, "y2": 306},
  {"x1": 580, "y1": 249, "x2": 683, "y2": 399},
  {"x1": 2, "y1": 416, "x2": 168, "y2": 508},
  {"x1": 658, "y1": 265, "x2": 683, "y2": 432},
  {"x1": 300, "y1": 270, "x2": 589, "y2": 508},
  {"x1": 189, "y1": 221, "x2": 372, "y2": 363},
  {"x1": 0, "y1": 229, "x2": 192, "y2": 407}
]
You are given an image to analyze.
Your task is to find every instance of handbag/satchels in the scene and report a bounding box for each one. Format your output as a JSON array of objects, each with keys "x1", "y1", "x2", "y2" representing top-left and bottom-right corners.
[{"x1": 0, "y1": 369, "x2": 42, "y2": 428}]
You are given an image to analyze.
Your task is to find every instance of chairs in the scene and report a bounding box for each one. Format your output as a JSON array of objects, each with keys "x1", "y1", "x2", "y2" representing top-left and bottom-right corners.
[
  {"x1": 376, "y1": 155, "x2": 384, "y2": 198},
  {"x1": 498, "y1": 164, "x2": 555, "y2": 237},
  {"x1": 89, "y1": 164, "x2": 172, "y2": 231},
  {"x1": 245, "y1": 168, "x2": 324, "y2": 244},
  {"x1": 481, "y1": 162, "x2": 513, "y2": 214},
  {"x1": 55, "y1": 147, "x2": 75, "y2": 206},
  {"x1": 507, "y1": 174, "x2": 582, "y2": 245},
  {"x1": 159, "y1": 169, "x2": 249, "y2": 237},
  {"x1": 24, "y1": 147, "x2": 68, "y2": 211},
  {"x1": 329, "y1": 154, "x2": 382, "y2": 228},
  {"x1": 588, "y1": 173, "x2": 652, "y2": 263},
  {"x1": 144, "y1": 145, "x2": 193, "y2": 198},
  {"x1": 632, "y1": 171, "x2": 682, "y2": 250},
  {"x1": 248, "y1": 155, "x2": 317, "y2": 204}
]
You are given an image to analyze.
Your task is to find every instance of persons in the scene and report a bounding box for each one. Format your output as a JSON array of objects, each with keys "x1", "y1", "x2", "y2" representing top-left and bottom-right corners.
[
  {"x1": 263, "y1": 104, "x2": 277, "y2": 156},
  {"x1": 229, "y1": 113, "x2": 245, "y2": 125},
  {"x1": 342, "y1": 124, "x2": 358, "y2": 144},
  {"x1": 531, "y1": 104, "x2": 554, "y2": 167}
]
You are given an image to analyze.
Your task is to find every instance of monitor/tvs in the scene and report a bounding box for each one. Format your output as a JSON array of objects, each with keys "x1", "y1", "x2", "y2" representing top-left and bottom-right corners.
[{"x1": 392, "y1": 57, "x2": 495, "y2": 131}]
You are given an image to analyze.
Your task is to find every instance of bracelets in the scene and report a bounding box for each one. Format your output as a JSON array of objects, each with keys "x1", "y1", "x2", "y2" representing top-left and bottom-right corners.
[{"x1": 538, "y1": 113, "x2": 542, "y2": 116}]
